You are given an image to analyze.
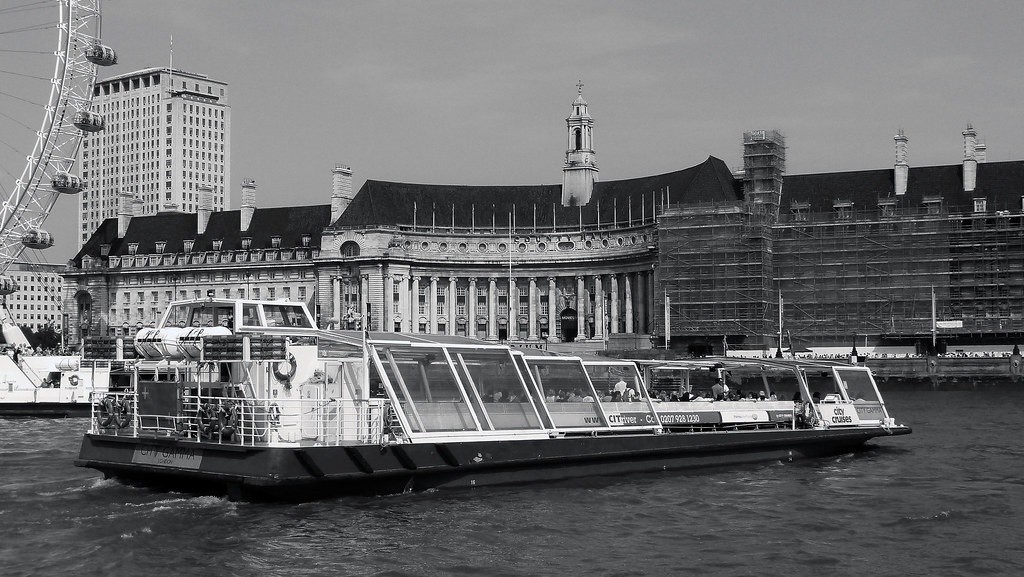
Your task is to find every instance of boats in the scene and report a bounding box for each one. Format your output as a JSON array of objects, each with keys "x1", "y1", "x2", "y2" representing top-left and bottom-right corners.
[{"x1": 0, "y1": 298, "x2": 913, "y2": 504}]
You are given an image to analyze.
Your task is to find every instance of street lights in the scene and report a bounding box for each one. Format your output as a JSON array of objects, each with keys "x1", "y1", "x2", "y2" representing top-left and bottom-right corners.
[
  {"x1": 244, "y1": 270, "x2": 251, "y2": 300},
  {"x1": 173, "y1": 275, "x2": 178, "y2": 326}
]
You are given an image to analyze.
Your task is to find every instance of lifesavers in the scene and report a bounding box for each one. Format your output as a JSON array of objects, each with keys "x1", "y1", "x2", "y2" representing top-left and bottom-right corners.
[
  {"x1": 217, "y1": 405, "x2": 239, "y2": 435},
  {"x1": 114, "y1": 399, "x2": 131, "y2": 429},
  {"x1": 272, "y1": 351, "x2": 298, "y2": 381},
  {"x1": 70, "y1": 375, "x2": 79, "y2": 386},
  {"x1": 196, "y1": 402, "x2": 216, "y2": 434},
  {"x1": 97, "y1": 399, "x2": 113, "y2": 427}
]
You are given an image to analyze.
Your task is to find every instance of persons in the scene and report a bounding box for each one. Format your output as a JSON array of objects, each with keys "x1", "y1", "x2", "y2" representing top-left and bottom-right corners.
[
  {"x1": 519, "y1": 344, "x2": 544, "y2": 350},
  {"x1": 0, "y1": 343, "x2": 67, "y2": 388},
  {"x1": 460, "y1": 387, "x2": 533, "y2": 403},
  {"x1": 545, "y1": 375, "x2": 823, "y2": 403},
  {"x1": 733, "y1": 351, "x2": 1011, "y2": 358}
]
